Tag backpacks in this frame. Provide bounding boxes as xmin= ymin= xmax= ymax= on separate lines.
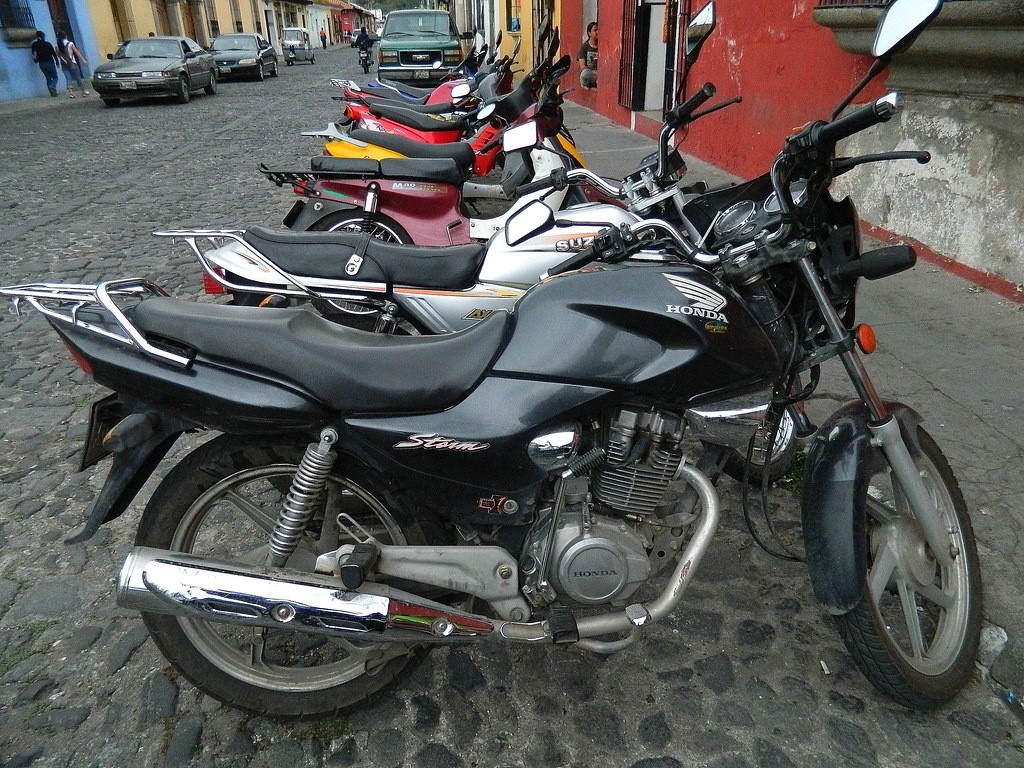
xmin=57 ymin=41 xmax=73 ymax=67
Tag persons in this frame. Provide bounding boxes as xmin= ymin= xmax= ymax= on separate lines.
xmin=576 ymin=22 xmax=598 ymax=88
xmin=320 ymin=28 xmax=327 ymax=49
xmin=345 ymin=29 xmax=352 ymax=43
xmin=31 ymin=31 xmax=59 ymax=97
xmin=54 ymin=29 xmax=89 ymax=98
xmin=356 ymin=26 xmax=374 ymax=64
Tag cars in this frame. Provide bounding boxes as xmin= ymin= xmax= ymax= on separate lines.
xmin=91 ymin=36 xmax=219 ymax=108
xmin=203 ymin=32 xmax=279 ymax=82
xmin=351 ymin=29 xmax=368 ymax=48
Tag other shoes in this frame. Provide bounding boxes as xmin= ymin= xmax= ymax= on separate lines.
xmin=83 ymin=91 xmax=89 ymax=97
xmin=50 ymin=90 xmax=59 ymax=97
xmin=70 ymin=94 xmax=76 ymax=98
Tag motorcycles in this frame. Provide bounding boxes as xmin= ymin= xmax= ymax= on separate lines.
xmin=278 ymin=27 xmax=315 ymax=66
xmin=150 ymin=1 xmax=802 ymax=488
xmin=355 ymin=42 xmax=375 ymax=74
xmin=0 ymin=0 xmax=983 ymax=718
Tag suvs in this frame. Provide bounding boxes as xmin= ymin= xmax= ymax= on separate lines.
xmin=368 ymin=8 xmax=474 ymax=87
